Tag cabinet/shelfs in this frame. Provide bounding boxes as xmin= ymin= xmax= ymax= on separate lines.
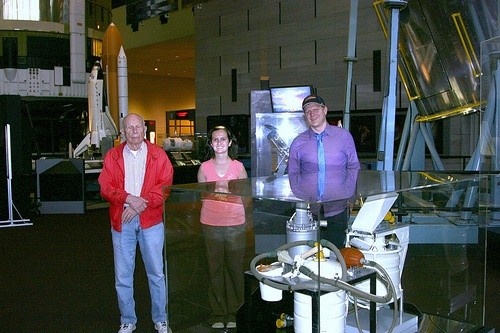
xmin=35 ymin=157 xmax=109 ymax=215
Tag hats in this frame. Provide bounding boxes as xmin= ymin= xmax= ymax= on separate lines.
xmin=302 ymin=95 xmax=325 ymax=109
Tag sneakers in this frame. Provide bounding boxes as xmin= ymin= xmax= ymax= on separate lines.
xmin=155 ymin=322 xmax=172 ymax=333
xmin=118 ymin=321 xmax=136 ymax=333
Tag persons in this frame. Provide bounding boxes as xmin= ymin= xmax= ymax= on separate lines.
xmin=98 ymin=112 xmax=174 ymax=333
xmin=198 ymin=125 xmax=248 ymax=328
xmin=288 ymin=94 xmax=361 ymax=250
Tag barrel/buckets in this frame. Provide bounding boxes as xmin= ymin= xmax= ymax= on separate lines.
xmin=256 ymin=265 xmax=282 ymax=301
xmin=285 ymin=202 xmax=318 ymax=260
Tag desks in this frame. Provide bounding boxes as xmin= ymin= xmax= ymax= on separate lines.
xmin=244 ymin=260 xmax=376 ymax=333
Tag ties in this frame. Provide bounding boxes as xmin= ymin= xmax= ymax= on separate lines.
xmin=315 ymin=133 xmax=328 ymax=196
